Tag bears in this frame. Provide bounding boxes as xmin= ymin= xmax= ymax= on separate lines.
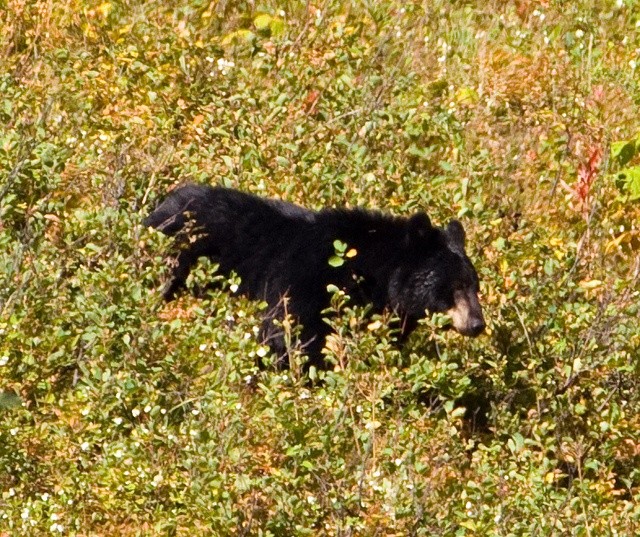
xmin=142 ymin=183 xmax=487 ymax=389
